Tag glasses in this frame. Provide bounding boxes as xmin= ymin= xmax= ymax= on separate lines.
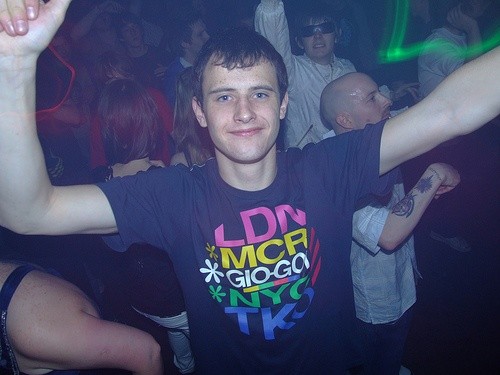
xmin=297 ymin=21 xmax=337 ymax=38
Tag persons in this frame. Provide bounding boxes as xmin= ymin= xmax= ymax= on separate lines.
xmin=67 ymin=0 xmax=215 ymax=101
xmin=0 ymin=0 xmax=500 ymax=375
xmin=320 ymin=73 xmax=460 ymax=375
xmin=0 ymin=264 xmax=162 ymax=375
xmin=254 ymin=1 xmax=421 ymax=150
xmin=416 ymin=0 xmax=487 ymax=101
xmin=92 ymin=79 xmax=195 ymax=375
xmin=169 ymin=69 xmax=213 ymax=168
